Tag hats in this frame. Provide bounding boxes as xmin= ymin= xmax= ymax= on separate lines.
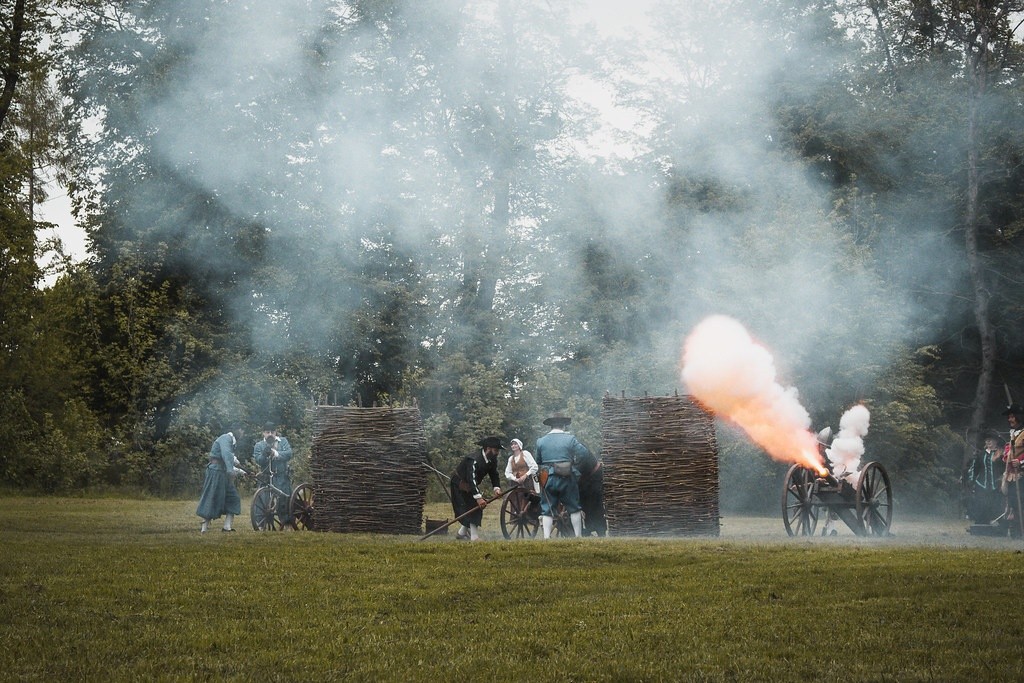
xmin=542 ymin=413 xmax=572 ymax=426
xmin=474 ymin=437 xmax=506 ymax=451
xmin=977 ymin=429 xmax=1006 ymax=448
xmin=263 ymin=421 xmax=277 ymax=431
xmin=1002 ymin=404 xmax=1024 ymax=416
xmin=817 ymin=427 xmax=833 ymax=449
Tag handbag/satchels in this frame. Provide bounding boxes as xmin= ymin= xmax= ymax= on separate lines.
xmin=554 ymin=462 xmax=571 ymax=478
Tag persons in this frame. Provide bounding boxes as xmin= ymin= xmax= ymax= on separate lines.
xmin=789 ymin=423 xmax=839 ymax=537
xmin=964 ymin=403 xmax=1024 ymax=540
xmin=196 ymin=421 xmax=293 ymax=533
xmin=449 ymin=415 xmax=604 ymax=540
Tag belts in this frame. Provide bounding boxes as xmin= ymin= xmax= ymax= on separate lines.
xmin=585 ymin=461 xmax=601 ymax=475
xmin=451 ymin=468 xmax=471 ymax=492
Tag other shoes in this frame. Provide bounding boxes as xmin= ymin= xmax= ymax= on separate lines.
xmin=456 ymin=532 xmax=469 ymax=540
xmin=822 ymin=526 xmax=826 ymax=535
xmin=832 ymin=530 xmax=837 ymax=535
xmin=221 ymin=527 xmax=236 ymax=531
